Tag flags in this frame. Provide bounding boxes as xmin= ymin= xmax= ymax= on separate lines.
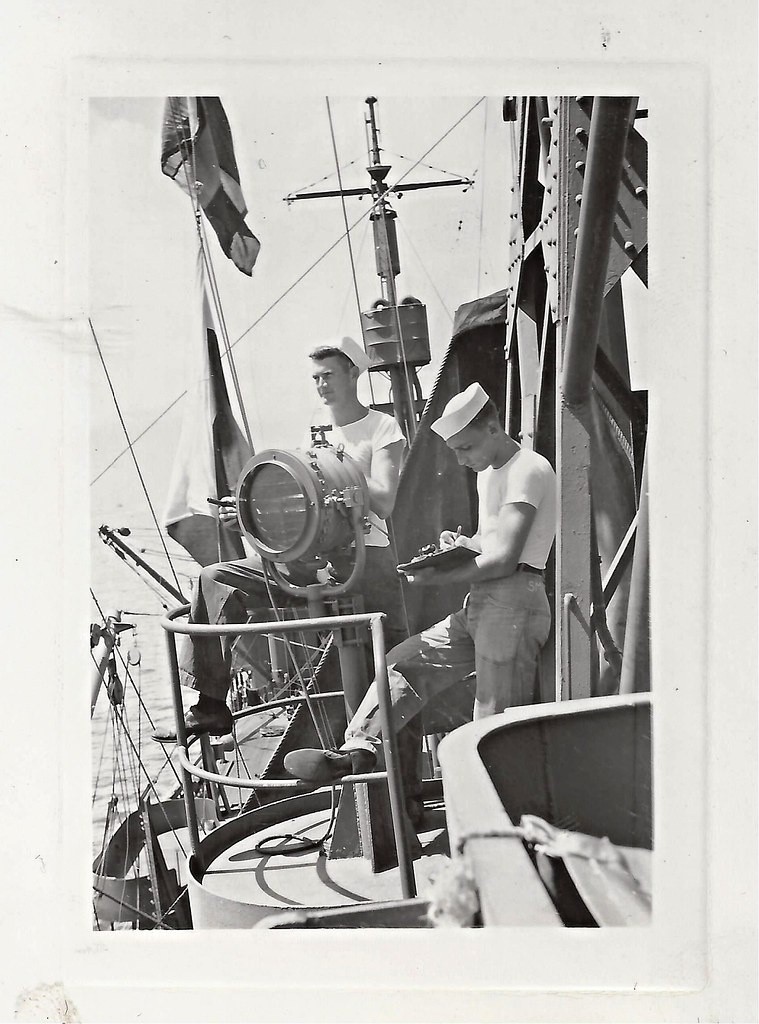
xmin=164 ymin=255 xmax=248 ymax=569
xmin=161 ymin=96 xmax=260 ymax=277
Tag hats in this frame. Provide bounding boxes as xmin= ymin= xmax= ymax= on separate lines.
xmin=317 ymin=335 xmax=371 ymax=377
xmin=429 ymin=382 xmax=490 ymax=440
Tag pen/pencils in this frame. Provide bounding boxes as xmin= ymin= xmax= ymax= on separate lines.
xmin=456 ymin=525 xmax=462 ymax=539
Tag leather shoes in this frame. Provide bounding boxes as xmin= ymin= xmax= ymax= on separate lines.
xmin=150 ymin=705 xmax=234 ymax=743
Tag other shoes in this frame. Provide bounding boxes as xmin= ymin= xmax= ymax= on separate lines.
xmin=284 ymin=749 xmax=377 ymax=781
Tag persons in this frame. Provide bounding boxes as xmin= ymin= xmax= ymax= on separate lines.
xmin=284 ymin=381 xmax=556 ymax=779
xmin=152 ymin=335 xmax=423 ymax=828
